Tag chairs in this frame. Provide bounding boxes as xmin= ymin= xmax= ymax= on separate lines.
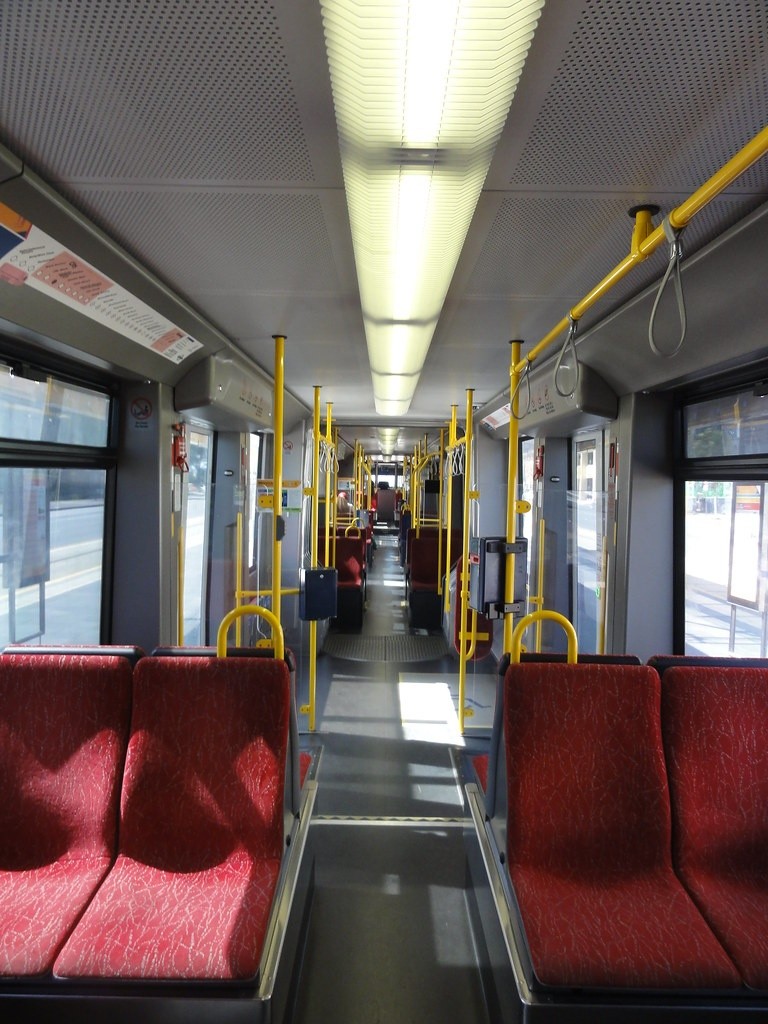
xmin=399 ymin=510 xmax=448 ymax=592
xmin=318 ymin=508 xmax=372 ymax=590
xmin=0 ymin=643 xmax=312 ymax=996
xmin=467 ymin=646 xmax=768 ymax=1003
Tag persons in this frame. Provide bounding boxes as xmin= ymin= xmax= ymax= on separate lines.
xmin=336 ymin=492 xmax=356 ymax=513
